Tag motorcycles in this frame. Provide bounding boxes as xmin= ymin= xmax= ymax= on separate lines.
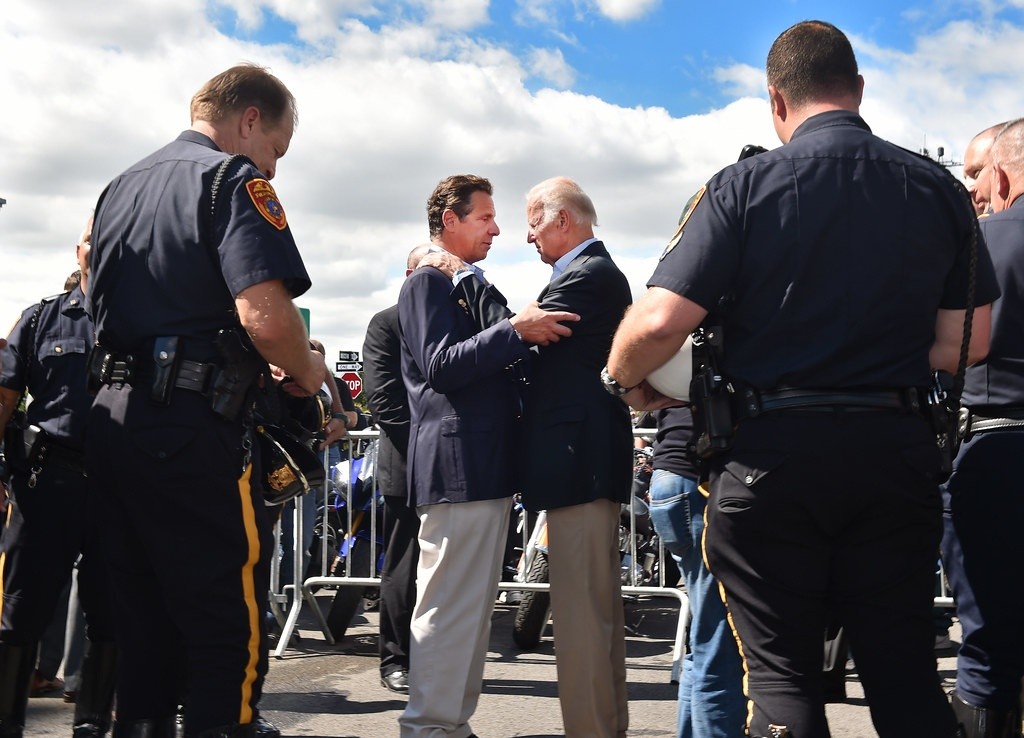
xmin=311 ymin=411 xmax=682 ymax=650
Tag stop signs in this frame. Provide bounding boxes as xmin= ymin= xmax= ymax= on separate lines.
xmin=342 ymin=373 xmax=363 ymax=399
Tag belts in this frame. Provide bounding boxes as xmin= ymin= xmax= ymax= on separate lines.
xmin=757 ymin=385 xmax=909 ymax=414
xmin=109 ymin=360 xmax=206 ymax=397
xmin=970 ymin=407 xmax=1024 ymax=433
xmin=49 ymin=447 xmax=90 ymax=477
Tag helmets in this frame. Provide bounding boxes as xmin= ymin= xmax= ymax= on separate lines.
xmin=259 ymin=424 xmax=326 ymax=507
xmin=280 ymin=376 xmax=334 ymax=435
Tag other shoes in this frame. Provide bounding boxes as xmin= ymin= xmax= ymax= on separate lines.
xmin=63 ymin=673 xmax=82 ymax=702
xmin=28 ymin=669 xmax=65 ymax=698
xmin=319 ymin=630 xmax=362 ymax=654
xmin=506 ymin=590 xmax=523 ymax=604
xmin=266 ymin=626 xmax=280 ymax=649
xmin=256 ymin=717 xmax=280 ymax=737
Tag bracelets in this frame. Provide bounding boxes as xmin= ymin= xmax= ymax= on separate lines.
xmin=333 ymin=402 xmax=343 ymax=406
xmin=513 ymin=329 xmax=523 ymax=341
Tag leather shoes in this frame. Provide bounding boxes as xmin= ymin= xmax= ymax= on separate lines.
xmin=381 ymin=669 xmax=409 ymax=694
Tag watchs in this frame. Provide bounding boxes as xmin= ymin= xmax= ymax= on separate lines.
xmin=600 ymin=366 xmax=646 ymax=397
xmin=452 ymin=267 xmax=473 ymax=279
xmin=331 ymin=414 xmax=349 ymax=428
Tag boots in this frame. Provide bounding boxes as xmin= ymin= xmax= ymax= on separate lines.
xmin=72 ymin=641 xmax=119 ymax=738
xmin=112 ymin=714 xmax=185 ymax=738
xmin=0 ymin=639 xmax=38 ymax=738
xmin=952 ymin=694 xmax=1023 ymax=738
xmin=193 ymin=721 xmax=256 ymax=738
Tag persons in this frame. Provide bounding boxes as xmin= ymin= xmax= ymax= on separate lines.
xmin=602 ymin=19 xmax=1023 ymax=738
xmin=363 ymin=175 xmax=634 ymax=738
xmin=0 ymin=66 xmax=362 ymax=738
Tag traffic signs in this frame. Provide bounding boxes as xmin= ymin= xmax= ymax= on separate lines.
xmin=335 ymin=351 xmax=363 ymax=372
